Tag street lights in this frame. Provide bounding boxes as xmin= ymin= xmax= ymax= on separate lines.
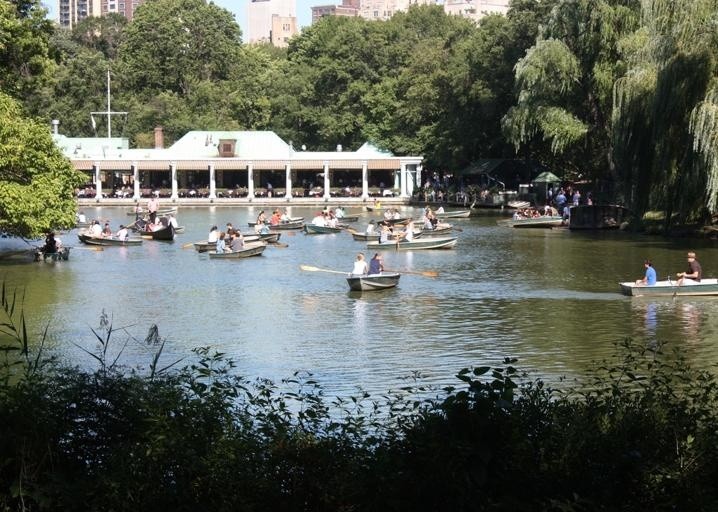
xmin=247 ymin=162 xmax=256 ymax=199
xmin=131 ymin=162 xmax=141 ymax=200
xmin=93 ymin=162 xmax=103 ymax=198
xmin=400 ymin=161 xmax=410 ymax=198
xmin=169 ymin=162 xmax=179 ymax=199
xmin=360 ymin=161 xmax=370 ymax=199
xmin=207 ymin=162 xmax=217 ymax=199
xmin=322 ymin=161 xmax=331 ymax=198
xmin=284 ymin=162 xmax=293 ymax=198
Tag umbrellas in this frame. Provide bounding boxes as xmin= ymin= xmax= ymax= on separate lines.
xmin=532 ymin=171 xmax=561 ymax=204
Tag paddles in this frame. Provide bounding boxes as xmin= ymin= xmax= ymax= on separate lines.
xmin=128 ymin=235 xmax=153 ymax=240
xmin=383 ymin=268 xmax=438 ymax=278
xmin=300 ymin=264 xmax=352 ymax=274
xmin=244 ymin=244 xmax=289 ymax=248
xmin=339 ymin=227 xmax=356 ymax=234
xmin=126 ymin=218 xmax=140 ymax=229
xmin=60 ymin=246 xmax=104 ymax=251
xmin=672 ymin=278 xmax=684 ymax=297
xmin=184 ymin=239 xmax=208 ymax=248
xmin=271 ymin=231 xmax=296 ymax=236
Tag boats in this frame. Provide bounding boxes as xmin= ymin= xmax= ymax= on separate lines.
xmin=75 ymin=221 xmax=94 ymax=228
xmin=623 ymin=295 xmax=718 ymax=309
xmin=135 ymin=217 xmax=175 ymax=241
xmin=332 ymin=214 xmax=360 ymax=222
xmin=366 ymin=206 xmax=394 ymax=211
xmin=174 ymin=227 xmax=185 ymax=235
xmin=242 ymin=233 xmax=281 ymax=242
xmin=434 ymin=210 xmax=471 ymax=218
xmin=494 ymin=217 xmax=563 ymax=229
xmin=208 ymin=244 xmax=267 ymax=259
xmin=249 ymin=214 xmax=305 ymax=229
xmin=351 ymin=218 xmax=459 ymax=249
xmin=127 ymin=206 xmax=179 ymax=218
xmin=77 ymin=234 xmax=144 ymax=247
xmin=303 ymin=222 xmax=350 ymax=234
xmin=617 ymin=278 xmax=718 ymax=297
xmin=34 ymin=247 xmax=71 ymax=263
xmin=194 ymin=235 xmax=262 ymax=251
xmin=345 ymin=273 xmax=400 ymax=291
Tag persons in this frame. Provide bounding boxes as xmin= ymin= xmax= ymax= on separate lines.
xmin=634 ymin=258 xmax=656 ymax=285
xmin=353 ymin=253 xmax=369 ymax=276
xmin=202 ymin=182 xmax=274 ymax=198
xmin=41 ymin=232 xmax=63 ymax=255
xmin=676 ymin=251 xmax=701 ymax=286
xmin=77 ymin=183 xmax=156 ymax=198
xmin=78 ymin=212 xmax=130 ymax=241
xmin=412 ymin=181 xmax=593 ymax=220
xmin=134 ymin=197 xmax=178 ymax=232
xmin=312 ymin=197 xmax=445 ymax=243
xmin=309 ymin=181 xmax=384 ymax=197
xmin=369 ymin=251 xmax=385 ymax=274
xmin=208 ymin=208 xmax=294 ymax=254
